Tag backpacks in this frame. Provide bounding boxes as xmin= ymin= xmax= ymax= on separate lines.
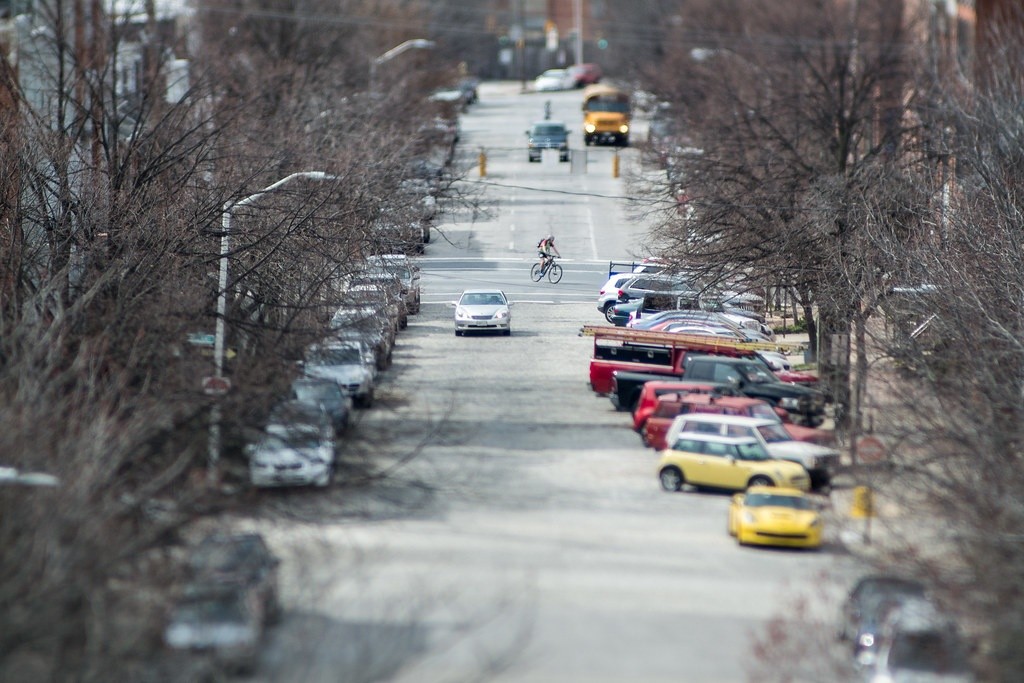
xmin=537 ymin=238 xmax=545 ymax=247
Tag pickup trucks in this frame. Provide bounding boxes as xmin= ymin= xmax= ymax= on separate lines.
xmin=607 ymin=352 xmax=826 ymax=416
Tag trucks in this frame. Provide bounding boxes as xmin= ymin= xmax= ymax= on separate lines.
xmin=580 ymin=83 xmax=636 ymax=148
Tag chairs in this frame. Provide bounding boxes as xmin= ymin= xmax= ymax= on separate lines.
xmin=487 ymin=296 xmax=503 ymax=304
xmin=464 ymin=296 xmax=476 ymax=303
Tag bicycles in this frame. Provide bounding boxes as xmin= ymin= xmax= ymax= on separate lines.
xmin=530 ymin=252 xmax=563 ymax=284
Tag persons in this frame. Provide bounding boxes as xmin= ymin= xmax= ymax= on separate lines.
xmin=538 ymin=234 xmax=560 ymax=277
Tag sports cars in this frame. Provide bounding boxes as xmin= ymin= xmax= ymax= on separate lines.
xmin=728 ymin=485 xmax=830 ymax=551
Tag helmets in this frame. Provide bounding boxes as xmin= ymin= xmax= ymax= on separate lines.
xmin=547 ymin=235 xmax=554 ymax=240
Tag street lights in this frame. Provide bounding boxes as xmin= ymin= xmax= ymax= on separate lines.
xmin=368 ymin=38 xmax=438 ymax=89
xmin=205 ymin=170 xmax=336 ymax=477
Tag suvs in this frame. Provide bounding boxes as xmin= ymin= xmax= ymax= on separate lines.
xmin=525 ymin=122 xmax=573 ymax=163
xmin=295 ymin=341 xmax=375 ymax=409
xmin=632 ymin=378 xmax=794 ymax=442
xmin=645 ymin=393 xmax=831 ymax=454
xmin=664 ymin=412 xmax=842 ymax=488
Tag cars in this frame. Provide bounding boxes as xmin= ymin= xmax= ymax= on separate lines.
xmin=533 ymin=62 xmax=602 ymax=92
xmin=588 ymin=253 xmax=820 ymax=405
xmin=339 ymin=75 xmax=477 ymax=335
xmin=322 ymin=305 xmax=394 ymax=371
xmin=834 ymin=571 xmax=978 ymax=683
xmin=450 ymin=288 xmax=515 ymax=337
xmin=655 ymin=431 xmax=813 ymax=497
xmin=258 ymin=398 xmax=340 ymax=445
xmin=157 ymin=531 xmax=285 ymax=677
xmin=242 ymin=423 xmax=336 ymax=490
xmin=880 ymin=263 xmax=947 ymax=306
xmin=274 ymin=378 xmax=353 ymax=439
